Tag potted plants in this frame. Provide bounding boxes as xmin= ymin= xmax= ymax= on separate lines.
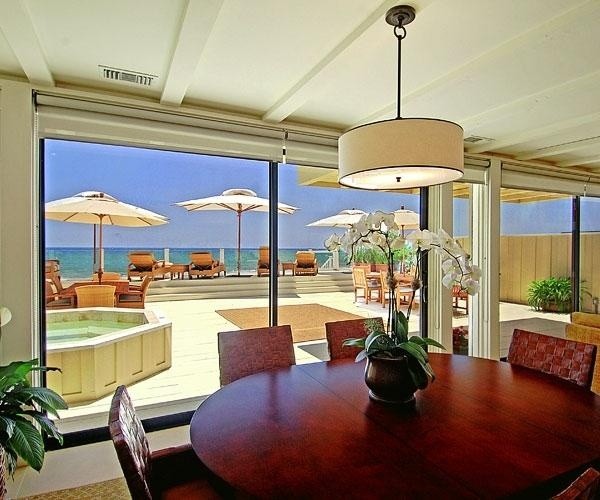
xmin=525 ymin=272 xmax=594 ymax=317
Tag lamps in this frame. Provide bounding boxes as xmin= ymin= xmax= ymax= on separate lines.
xmin=328 ymin=3 xmax=466 ymax=192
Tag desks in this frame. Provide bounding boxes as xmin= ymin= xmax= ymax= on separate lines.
xmin=169 ymin=264 xmax=190 ymax=281
xmin=281 ymin=262 xmax=295 ymax=277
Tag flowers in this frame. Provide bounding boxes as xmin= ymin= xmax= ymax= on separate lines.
xmin=316 ymin=205 xmax=484 ymax=392
xmin=0 ymin=303 xmax=71 ymax=486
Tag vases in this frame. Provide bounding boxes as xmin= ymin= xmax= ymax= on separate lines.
xmin=360 ymin=345 xmax=421 ymax=409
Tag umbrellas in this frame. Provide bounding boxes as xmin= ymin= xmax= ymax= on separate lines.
xmin=169 ymin=189 xmax=301 ymax=276
xmin=305 ymin=205 xmax=420 ymax=301
xmin=46 ymin=190 xmax=172 ymax=285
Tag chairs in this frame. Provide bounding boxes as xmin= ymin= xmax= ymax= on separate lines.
xmin=103 ymin=382 xmax=246 ymax=500
xmin=353 ymin=260 xmax=423 ymax=314
xmin=186 ymin=250 xmax=227 ymax=282
xmin=540 ymin=465 xmax=600 ymax=500
xmin=504 ymin=327 xmax=598 ymax=394
xmin=125 ymin=249 xmax=161 ymax=282
xmin=255 ymin=245 xmax=273 ymax=278
xmin=45 ymin=257 xmax=60 ymax=272
xmin=45 ymin=268 xmax=153 ymax=312
xmin=214 ymin=322 xmax=296 ymax=390
xmin=295 ymin=251 xmax=318 ymax=276
xmin=563 ymin=310 xmax=600 ymax=397
xmin=322 ymin=312 xmax=391 ymax=363
